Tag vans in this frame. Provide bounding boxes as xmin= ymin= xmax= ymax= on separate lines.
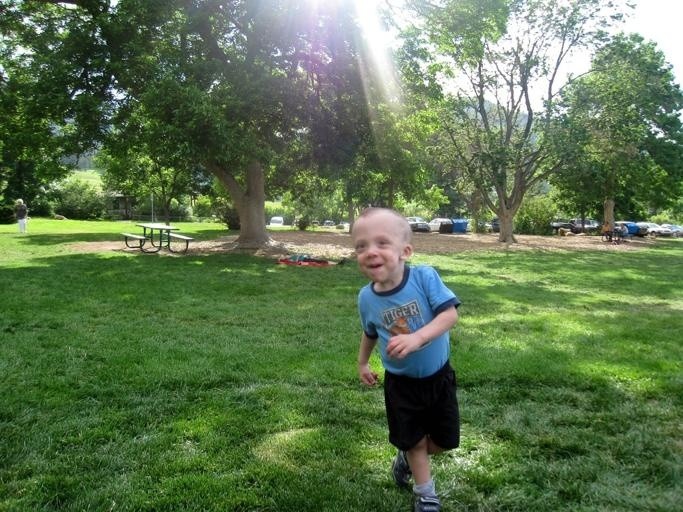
xmin=268 ymin=215 xmax=286 ymax=227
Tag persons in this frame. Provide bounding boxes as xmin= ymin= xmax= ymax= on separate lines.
xmin=348 ymin=207 xmax=461 ymax=511
xmin=617 ymin=223 xmax=628 ymax=241
xmin=602 ymin=221 xmax=612 ymax=242
xmin=13 ymin=198 xmax=28 ymax=234
xmin=279 ymin=253 xmax=346 ymax=267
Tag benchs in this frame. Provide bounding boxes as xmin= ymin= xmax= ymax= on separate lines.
xmin=120 ymin=223 xmax=195 ymax=253
xmin=603 ymin=230 xmax=633 ymax=242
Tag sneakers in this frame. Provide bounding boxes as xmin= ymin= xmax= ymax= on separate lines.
xmin=390 ymin=448 xmax=412 ymax=486
xmin=415 ymin=492 xmax=440 ymax=511
